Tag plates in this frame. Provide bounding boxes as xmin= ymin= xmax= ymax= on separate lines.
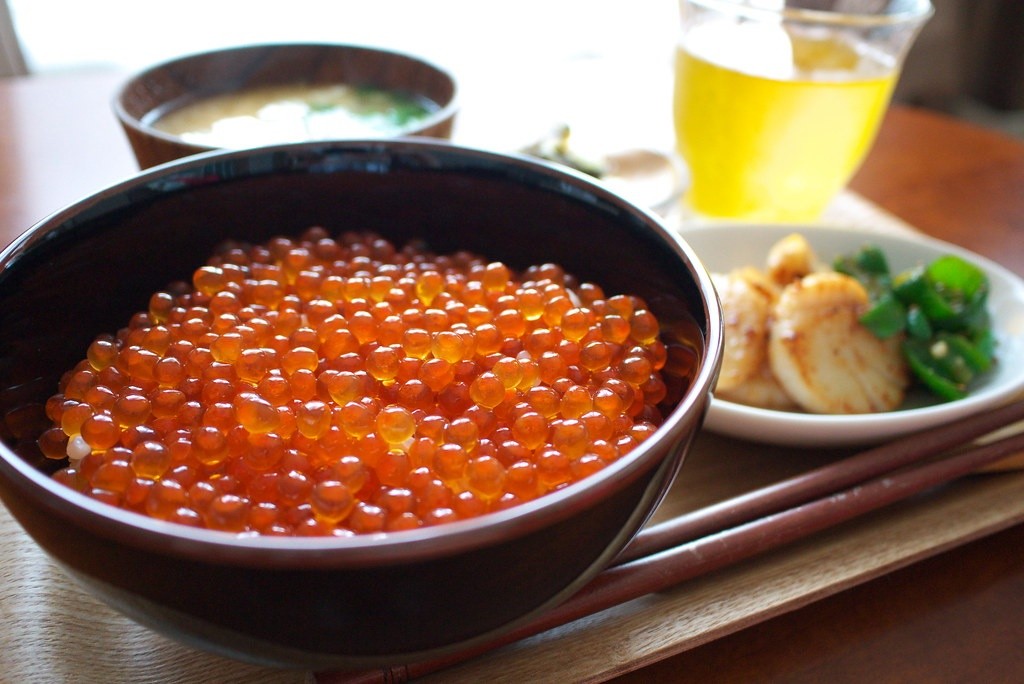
xmin=675 ymin=223 xmax=1023 ymax=445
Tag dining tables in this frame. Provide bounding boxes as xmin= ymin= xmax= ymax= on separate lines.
xmin=1 ymin=60 xmax=1024 ymax=684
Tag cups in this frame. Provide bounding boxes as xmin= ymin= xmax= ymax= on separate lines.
xmin=672 ymin=1 xmax=935 ymax=222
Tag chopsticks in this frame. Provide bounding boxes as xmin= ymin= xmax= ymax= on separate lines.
xmin=311 ymin=394 xmax=1024 ymax=684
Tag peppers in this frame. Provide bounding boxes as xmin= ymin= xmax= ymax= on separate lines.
xmin=834 ymin=245 xmax=993 ymax=399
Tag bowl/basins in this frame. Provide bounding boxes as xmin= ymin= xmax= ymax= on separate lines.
xmin=0 ymin=137 xmax=723 ymax=669
xmin=113 ymin=42 xmax=460 ymax=173
